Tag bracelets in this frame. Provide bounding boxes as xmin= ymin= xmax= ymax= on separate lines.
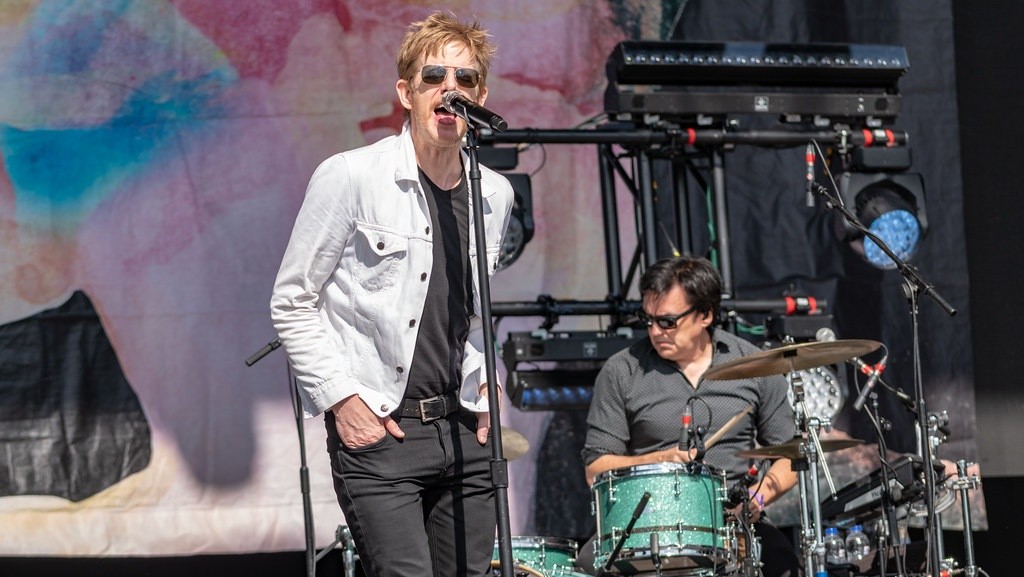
xmin=749 ymin=489 xmax=765 ymax=512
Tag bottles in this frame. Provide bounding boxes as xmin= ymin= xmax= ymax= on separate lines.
xmin=846 ymin=525 xmax=869 ymax=577
xmin=824 ymin=528 xmax=846 ymax=564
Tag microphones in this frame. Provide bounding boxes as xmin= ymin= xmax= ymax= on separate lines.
xmin=724 ymin=459 xmax=765 ymax=509
xmin=816 ymin=327 xmax=875 ymax=378
xmin=679 ymin=403 xmax=692 ymax=452
xmin=442 ymin=90 xmax=508 ymax=133
xmin=853 ymin=355 xmax=888 ymax=411
xmin=804 ymin=142 xmax=816 ymax=208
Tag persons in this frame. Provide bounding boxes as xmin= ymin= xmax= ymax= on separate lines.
xmin=574 ymin=254 xmax=799 ymax=577
xmin=270 ymin=8 xmax=514 ymax=577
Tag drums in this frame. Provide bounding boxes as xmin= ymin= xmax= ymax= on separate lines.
xmin=591 ymin=461 xmax=729 ymax=577
xmin=491 ymin=538 xmax=579 ymax=576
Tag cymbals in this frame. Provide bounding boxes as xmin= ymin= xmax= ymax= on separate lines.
xmin=737 ymin=436 xmax=865 ymax=460
xmin=489 ymin=426 xmax=530 ymax=462
xmin=699 ymin=339 xmax=883 ymax=382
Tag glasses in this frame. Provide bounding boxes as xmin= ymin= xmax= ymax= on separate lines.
xmin=638 ymin=306 xmax=697 ymax=329
xmin=417 ymin=65 xmax=482 ymax=88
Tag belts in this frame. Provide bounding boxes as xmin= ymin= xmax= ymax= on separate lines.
xmin=391 ymin=389 xmax=460 ymax=423
